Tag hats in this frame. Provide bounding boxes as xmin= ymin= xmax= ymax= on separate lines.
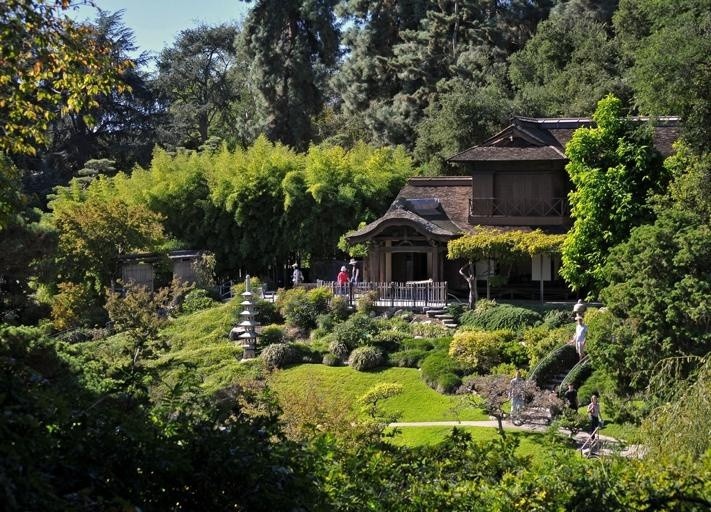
xmin=340 ymin=266 xmax=346 ymax=271
xmin=348 ymin=259 xmax=357 ymax=264
xmin=291 ymin=264 xmax=297 ymax=267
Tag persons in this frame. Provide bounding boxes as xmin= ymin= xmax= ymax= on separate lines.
xmin=349 ymin=259 xmax=360 ymax=285
xmin=587 ymin=395 xmax=603 ymax=441
xmin=291 ymin=263 xmax=305 ymax=284
xmin=509 ymin=371 xmax=525 ymax=420
xmin=550 ymin=385 xmax=562 ymax=420
xmin=568 ymin=313 xmax=588 ymax=360
xmin=565 ymin=382 xmax=580 ymax=414
xmin=338 ymin=266 xmax=351 ymax=285
